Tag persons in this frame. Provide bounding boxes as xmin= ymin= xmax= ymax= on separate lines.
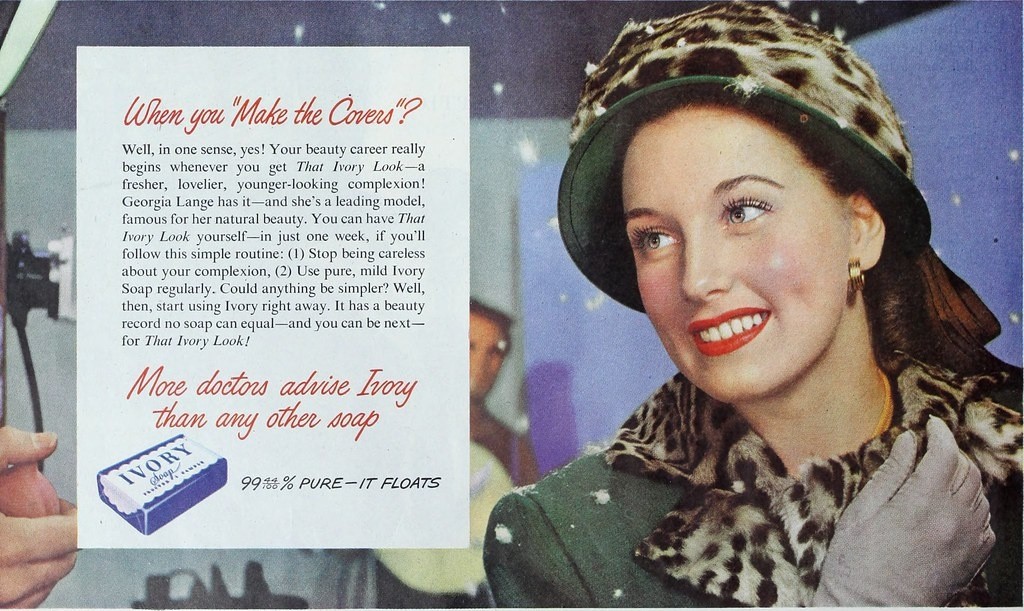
xmin=483 ymin=0 xmax=1023 ymax=608
xmin=298 ymin=300 xmax=534 ymax=609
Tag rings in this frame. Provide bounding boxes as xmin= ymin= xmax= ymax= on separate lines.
xmin=8 ymin=465 xmax=13 ymax=475
